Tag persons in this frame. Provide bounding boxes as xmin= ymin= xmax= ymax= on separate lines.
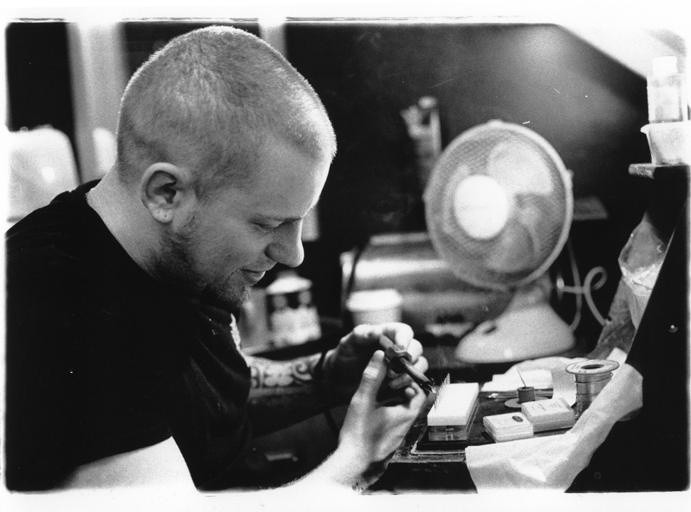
xmin=6 ymin=23 xmax=434 ymax=494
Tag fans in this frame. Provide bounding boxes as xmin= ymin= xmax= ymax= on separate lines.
xmin=421 ymin=118 xmax=588 ymax=364
